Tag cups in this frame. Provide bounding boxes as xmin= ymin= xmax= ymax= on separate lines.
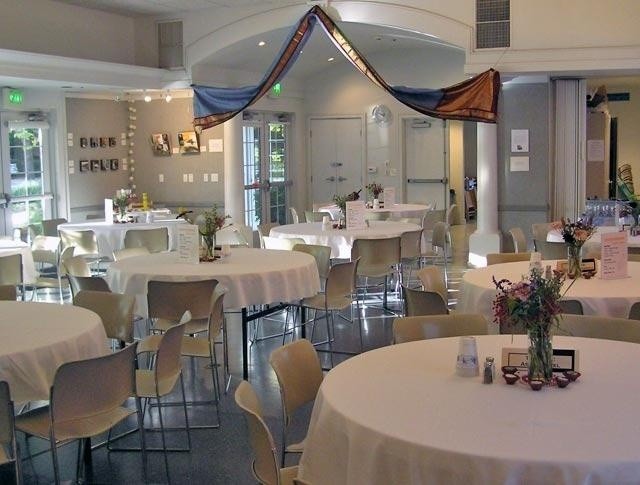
xmin=146 ymin=210 xmax=152 ymax=225
xmin=560 ymin=269 xmax=597 ymax=279
xmin=221 ymin=242 xmax=231 ymax=257
xmin=142 ymin=192 xmax=148 ymax=208
xmin=502 ymin=365 xmax=581 ymax=390
xmin=323 ymin=216 xmax=330 ymax=226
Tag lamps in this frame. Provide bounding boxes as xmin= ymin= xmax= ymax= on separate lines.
xmin=110 ymin=87 xmax=174 ymax=104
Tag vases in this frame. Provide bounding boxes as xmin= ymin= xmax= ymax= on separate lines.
xmin=526 ymin=329 xmax=554 ymax=386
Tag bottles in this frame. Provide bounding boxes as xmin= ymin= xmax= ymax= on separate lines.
xmin=482 ymin=357 xmax=497 ymax=384
xmin=455 ymin=336 xmax=479 ymax=377
xmin=332 ymin=218 xmax=346 ymax=229
xmin=545 ymin=265 xmax=552 ymax=280
xmin=366 ymin=198 xmax=380 ymax=209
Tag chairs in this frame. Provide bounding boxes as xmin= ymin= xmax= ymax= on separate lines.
xmin=1 ymin=379 xmax=37 ymax=483
xmin=106 ymin=310 xmax=195 ymax=454
xmin=282 ymin=254 xmax=365 ymax=372
xmin=2 ymin=201 xmax=637 ymax=322
xmin=234 ymin=381 xmax=305 ymax=483
xmin=135 ymin=291 xmax=225 ymax=434
xmin=268 ymin=339 xmax=330 ymax=468
xmin=547 ymin=311 xmax=638 ymax=342
xmin=11 ymin=338 xmax=150 ymax=484
xmin=391 ymin=314 xmax=489 ymax=346
xmin=72 ymin=291 xmax=147 ymax=451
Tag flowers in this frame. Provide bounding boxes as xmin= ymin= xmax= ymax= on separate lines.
xmin=489 ymin=262 xmax=586 ymax=341
